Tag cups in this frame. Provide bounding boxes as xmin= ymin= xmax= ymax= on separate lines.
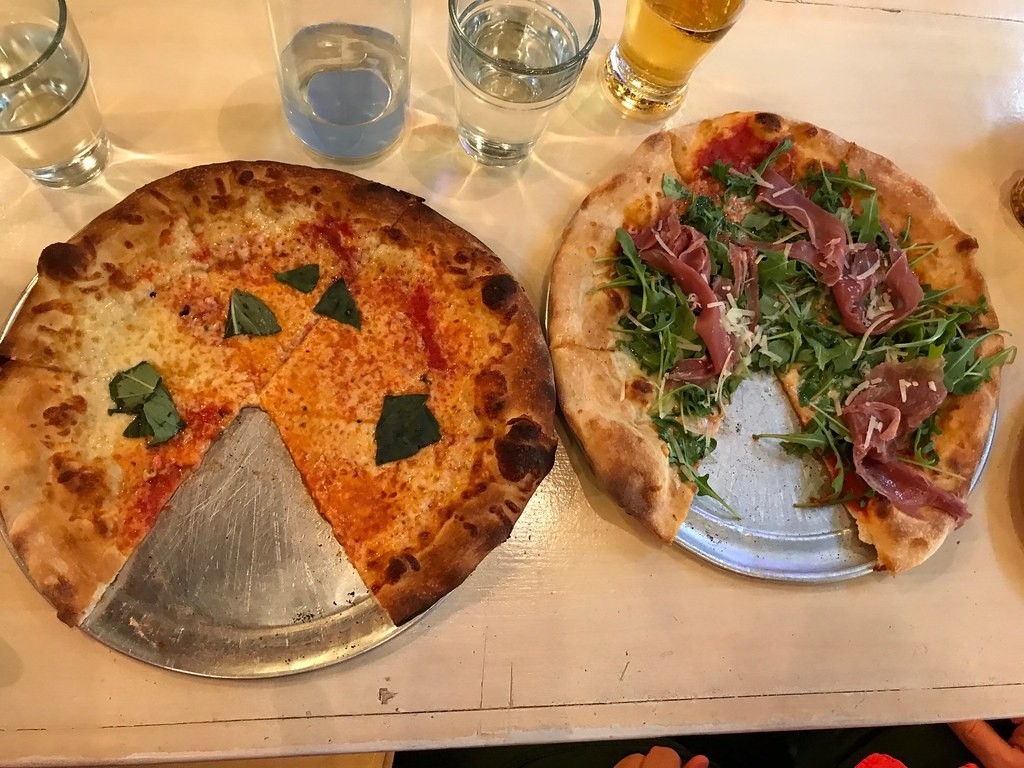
xmin=0 ymin=0 xmax=110 ymax=189
xmin=266 ymin=0 xmax=412 ymax=168
xmin=449 ymin=0 xmax=601 ymax=168
xmin=598 ymin=0 xmax=748 ymax=124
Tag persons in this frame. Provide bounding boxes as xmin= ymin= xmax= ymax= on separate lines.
xmin=385 ymin=718 xmax=1024 ymax=768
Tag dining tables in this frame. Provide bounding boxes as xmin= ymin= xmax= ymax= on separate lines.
xmin=0 ymin=1 xmax=1024 ymax=768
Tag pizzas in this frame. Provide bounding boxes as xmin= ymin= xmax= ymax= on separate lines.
xmin=548 ymin=114 xmax=1002 ymax=570
xmin=0 ymin=159 xmax=559 ymax=626
